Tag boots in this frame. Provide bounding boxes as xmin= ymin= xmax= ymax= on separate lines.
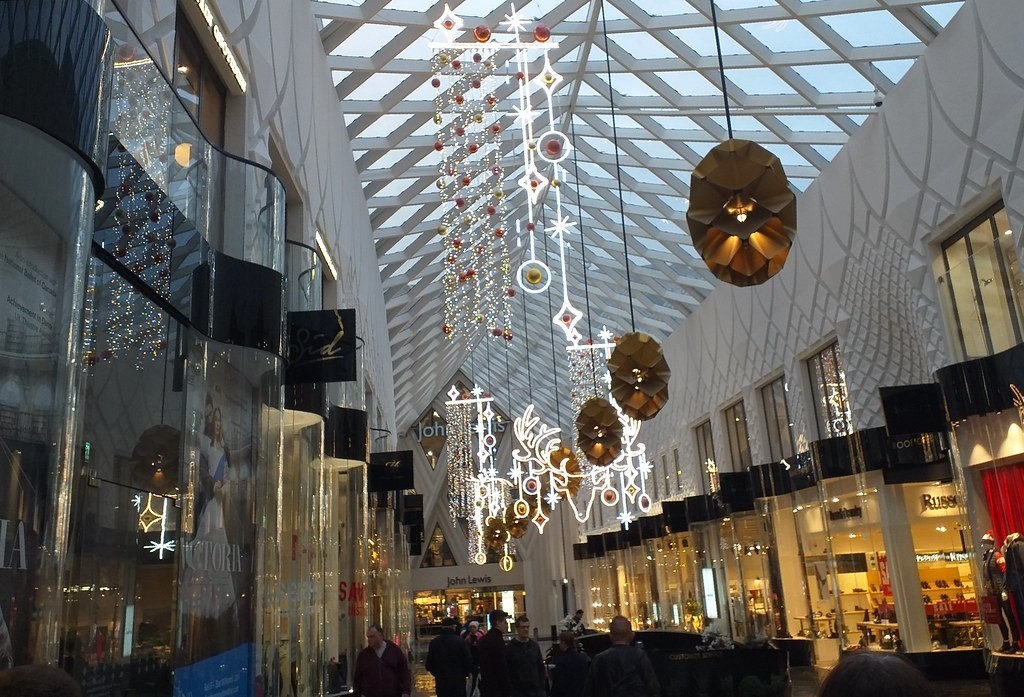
xmin=998 ymin=641 xmax=1010 ymax=652
xmin=1005 ymin=641 xmax=1019 ymax=654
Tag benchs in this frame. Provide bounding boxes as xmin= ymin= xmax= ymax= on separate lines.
xmin=857 ymin=621 xmax=899 ymax=649
xmin=793 ymin=616 xmax=837 ymax=638
xmin=948 ymin=620 xmax=982 ymax=649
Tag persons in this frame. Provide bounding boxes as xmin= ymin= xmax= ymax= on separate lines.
xmin=585 ymin=616 xmax=660 ymax=697
xmin=353 ymin=624 xmax=412 ymax=697
xmin=424 ymin=618 xmax=474 ymax=697
xmin=550 ymin=631 xmax=590 ymax=697
xmin=981 ymin=533 xmax=1021 ymax=654
xmin=458 ymin=615 xmax=485 ymax=637
xmin=1001 ymin=532 xmax=1024 ymax=655
xmin=465 ymin=621 xmax=484 ymax=697
xmin=477 ymin=610 xmax=511 ymax=697
xmin=181 ymin=406 xmax=240 ymax=647
xmin=504 ymin=615 xmax=550 ymax=697
xmin=563 ymin=609 xmax=586 ymax=636
xmin=701 ymin=616 xmax=716 ymax=636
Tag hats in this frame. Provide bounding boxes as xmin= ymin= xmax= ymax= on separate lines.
xmin=468 ymin=621 xmax=479 ymax=630
xmin=443 ymin=618 xmax=458 ymax=626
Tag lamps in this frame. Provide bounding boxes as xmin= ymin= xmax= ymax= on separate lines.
xmin=470 ymin=351 xmax=503 ymax=558
xmin=743 ymin=545 xmax=752 ymax=556
xmin=130 ymin=159 xmax=181 ymax=494
xmin=540 ymin=204 xmax=581 ymax=501
xmin=520 ymin=259 xmax=552 ymax=521
xmin=571 ymin=111 xmax=623 ymax=467
xmin=485 ymin=330 xmax=508 ymax=548
xmin=682 ymin=539 xmax=689 ymax=548
xmin=599 ymin=0 xmax=672 ymax=420
xmin=502 ymin=301 xmax=530 ymax=539
xmin=685 ymin=0 xmax=797 ymax=287
xmin=668 ymin=541 xmax=674 ymax=551
xmin=657 ymin=543 xmax=662 ymax=552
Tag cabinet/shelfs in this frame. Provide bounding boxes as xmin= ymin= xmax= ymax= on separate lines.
xmin=866 ymin=567 xmax=971 ymax=619
